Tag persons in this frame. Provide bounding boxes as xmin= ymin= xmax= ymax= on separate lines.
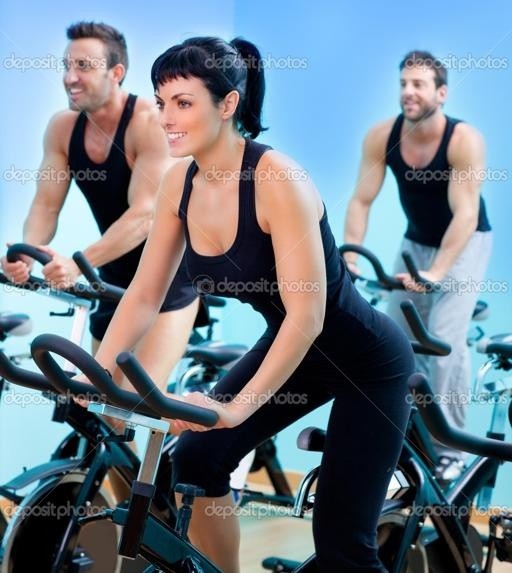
xmin=344 ymin=51 xmax=493 ymax=480
xmin=67 ymin=35 xmax=415 ymax=570
xmin=2 ymin=22 xmax=206 ymax=502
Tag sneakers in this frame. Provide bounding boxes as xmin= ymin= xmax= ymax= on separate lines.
xmin=435 ymin=456 xmax=463 ymax=481
